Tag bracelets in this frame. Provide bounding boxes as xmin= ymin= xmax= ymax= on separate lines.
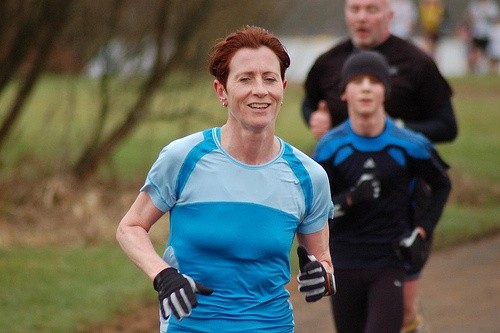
xmin=317 ymin=258 xmax=333 ymax=268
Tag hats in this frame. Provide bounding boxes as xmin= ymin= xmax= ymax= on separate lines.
xmin=338 ymin=50 xmax=392 ymax=100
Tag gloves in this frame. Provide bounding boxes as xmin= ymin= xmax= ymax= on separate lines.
xmin=395 ymin=227 xmax=423 ymax=272
xmin=152 ymin=266 xmax=215 ymax=322
xmin=295 ymin=245 xmax=329 ymax=302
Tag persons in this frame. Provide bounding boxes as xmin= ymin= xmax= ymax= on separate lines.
xmin=311 ymin=51 xmax=451 ymax=333
xmin=387 ymin=0 xmax=500 ymax=76
xmin=302 ymin=0 xmax=458 ymax=333
xmin=116 ymin=26 xmax=336 ymax=333
